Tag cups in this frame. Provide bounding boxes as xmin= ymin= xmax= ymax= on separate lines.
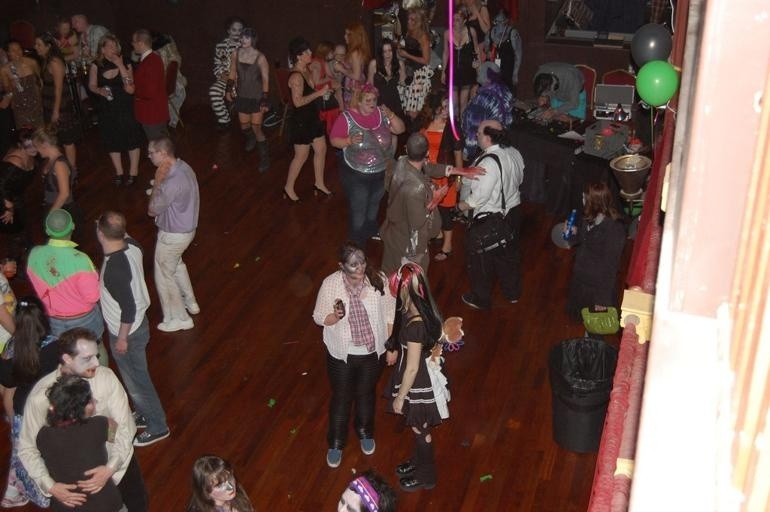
xmin=332 ymin=299 xmax=345 ymax=320
xmin=355 ymin=129 xmax=364 ymax=147
xmin=323 ymin=94 xmax=330 ymax=101
xmin=455 ymin=175 xmax=461 ymax=192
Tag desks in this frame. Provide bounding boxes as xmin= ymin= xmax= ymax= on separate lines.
xmin=509 ymin=106 xmax=616 ymax=225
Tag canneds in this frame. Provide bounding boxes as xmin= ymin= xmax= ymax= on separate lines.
xmin=103 ymin=86 xmax=113 ymax=101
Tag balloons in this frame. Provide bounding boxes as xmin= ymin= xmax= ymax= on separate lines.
xmin=635 ymin=60 xmax=678 ymax=109
xmin=630 ymin=21 xmax=673 ymax=67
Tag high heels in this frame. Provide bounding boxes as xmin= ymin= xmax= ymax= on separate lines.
xmin=282 ymin=186 xmax=304 ymax=205
xmin=313 ymin=184 xmax=334 ymax=200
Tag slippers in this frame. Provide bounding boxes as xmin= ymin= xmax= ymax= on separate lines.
xmin=433 ymin=250 xmax=454 ymax=263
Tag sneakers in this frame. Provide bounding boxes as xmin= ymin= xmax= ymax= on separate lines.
xmin=360 ymin=439 xmax=377 ymax=455
xmin=327 ymin=449 xmax=343 ymax=468
xmin=155 ymin=301 xmax=200 ymax=331
xmin=461 ymin=292 xmax=489 ymax=312
xmin=399 ymin=474 xmax=437 ymax=492
xmin=113 ymin=174 xmax=139 ymax=189
xmin=395 ymin=460 xmax=416 ymax=476
xmin=134 ymin=427 xmax=171 ymax=445
xmin=133 ymin=410 xmax=146 ymax=426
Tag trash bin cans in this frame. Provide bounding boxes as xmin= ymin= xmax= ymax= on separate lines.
xmin=548 ymin=339 xmax=618 ymax=453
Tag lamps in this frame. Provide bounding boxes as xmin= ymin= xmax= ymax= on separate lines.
xmin=609 ymin=152 xmax=653 ymax=218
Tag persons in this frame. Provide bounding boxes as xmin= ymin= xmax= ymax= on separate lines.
xmin=0 ymin=13 xmax=202 ymax=512
xmin=310 ymin=239 xmax=391 ymax=468
xmin=223 ymin=29 xmax=272 ymax=174
xmin=286 ymin=2 xmax=525 ymax=310
xmin=182 ymin=455 xmax=255 ymax=511
xmin=334 ymin=470 xmax=401 ymax=510
xmin=532 ymin=61 xmax=587 ymax=120
xmin=560 ymin=177 xmax=622 ymax=327
xmin=208 ymin=18 xmax=253 ymax=130
xmin=383 ymin=262 xmax=450 ymax=492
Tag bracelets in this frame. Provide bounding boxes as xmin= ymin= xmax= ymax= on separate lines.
xmin=396 ymin=393 xmax=407 ymax=400
xmin=261 ymin=92 xmax=271 ymax=107
xmin=225 ymin=79 xmax=235 ymax=94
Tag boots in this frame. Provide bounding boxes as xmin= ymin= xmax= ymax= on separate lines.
xmin=243 ymin=128 xmax=271 ymax=174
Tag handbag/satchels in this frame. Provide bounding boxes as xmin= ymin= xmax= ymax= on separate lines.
xmin=581 ymin=303 xmax=620 ymax=336
xmin=463 ymin=212 xmax=522 ymax=283
xmin=376 ymin=190 xmax=390 ymax=225
xmin=383 ymin=160 xmax=396 ymax=189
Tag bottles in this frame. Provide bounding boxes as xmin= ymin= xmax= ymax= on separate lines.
xmin=81 ymin=58 xmax=89 ymax=80
xmin=124 ymin=76 xmax=133 ymax=86
xmin=104 ymin=85 xmax=115 ymax=102
xmin=561 ymin=209 xmax=576 ymax=242
xmin=614 ymin=103 xmax=627 ymax=122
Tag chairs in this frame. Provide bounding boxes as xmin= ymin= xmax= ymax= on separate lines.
xmin=603 ymin=68 xmax=637 ymax=88
xmin=275 ymin=70 xmax=295 ymax=134
xmin=574 ymin=63 xmax=596 ymax=110
